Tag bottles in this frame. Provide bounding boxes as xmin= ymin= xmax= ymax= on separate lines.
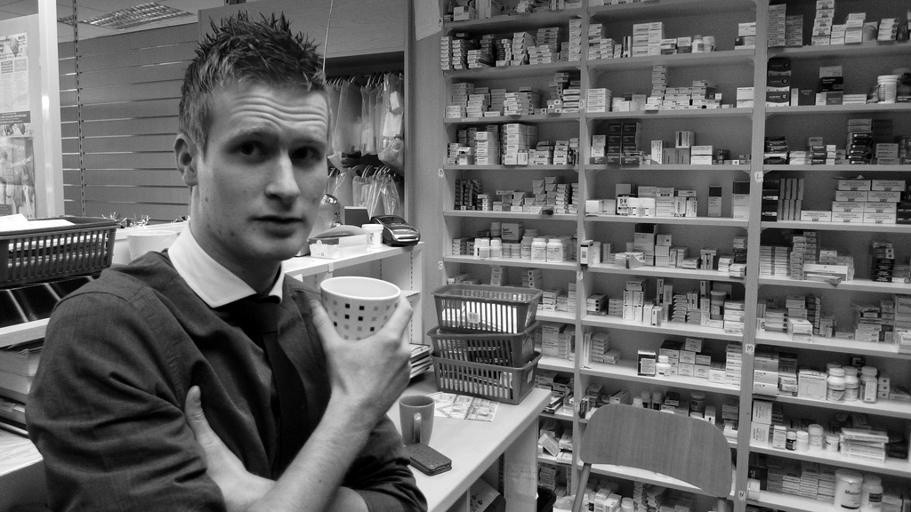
xmin=691 ymin=34 xmax=717 ymax=52
xmin=630 ymin=389 xmax=708 ymax=418
xmin=833 ymin=469 xmax=885 ymax=511
xmin=619 ymin=497 xmax=635 ymax=511
xmin=471 ymin=219 xmax=576 ymax=263
xmin=823 ymin=366 xmax=882 ymax=406
xmin=783 ymin=424 xmax=842 ymax=458
xmin=654 ymin=356 xmax=673 ymax=379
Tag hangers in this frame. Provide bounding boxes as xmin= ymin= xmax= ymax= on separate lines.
xmin=324 ymin=71 xmax=403 ymax=88
xmin=326 ymin=160 xmax=397 ymax=180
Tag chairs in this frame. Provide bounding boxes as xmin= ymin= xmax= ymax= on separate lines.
xmin=570 ymin=404 xmax=733 ymax=512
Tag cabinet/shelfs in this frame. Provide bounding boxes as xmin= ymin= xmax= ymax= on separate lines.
xmin=408 ymin=0 xmax=911 ymax=511
xmin=0 ymin=224 xmax=428 ymax=479
xmin=199 ymin=0 xmax=412 ymax=228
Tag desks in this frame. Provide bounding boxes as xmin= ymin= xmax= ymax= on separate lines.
xmin=385 ymin=371 xmax=553 ymax=511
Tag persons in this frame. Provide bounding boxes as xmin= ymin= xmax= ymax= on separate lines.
xmin=25 ymin=7 xmax=430 ymax=512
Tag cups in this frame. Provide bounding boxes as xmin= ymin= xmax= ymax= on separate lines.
xmin=399 ymin=393 xmax=435 ymax=447
xmin=316 ymin=275 xmax=401 ymax=342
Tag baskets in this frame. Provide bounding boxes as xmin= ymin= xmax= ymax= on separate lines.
xmin=431 ymin=283 xmax=544 ymax=337
xmin=429 ymin=349 xmax=544 ymax=406
xmin=425 ymin=319 xmax=542 ymax=368
xmin=0 ymin=216 xmax=120 ymax=289
